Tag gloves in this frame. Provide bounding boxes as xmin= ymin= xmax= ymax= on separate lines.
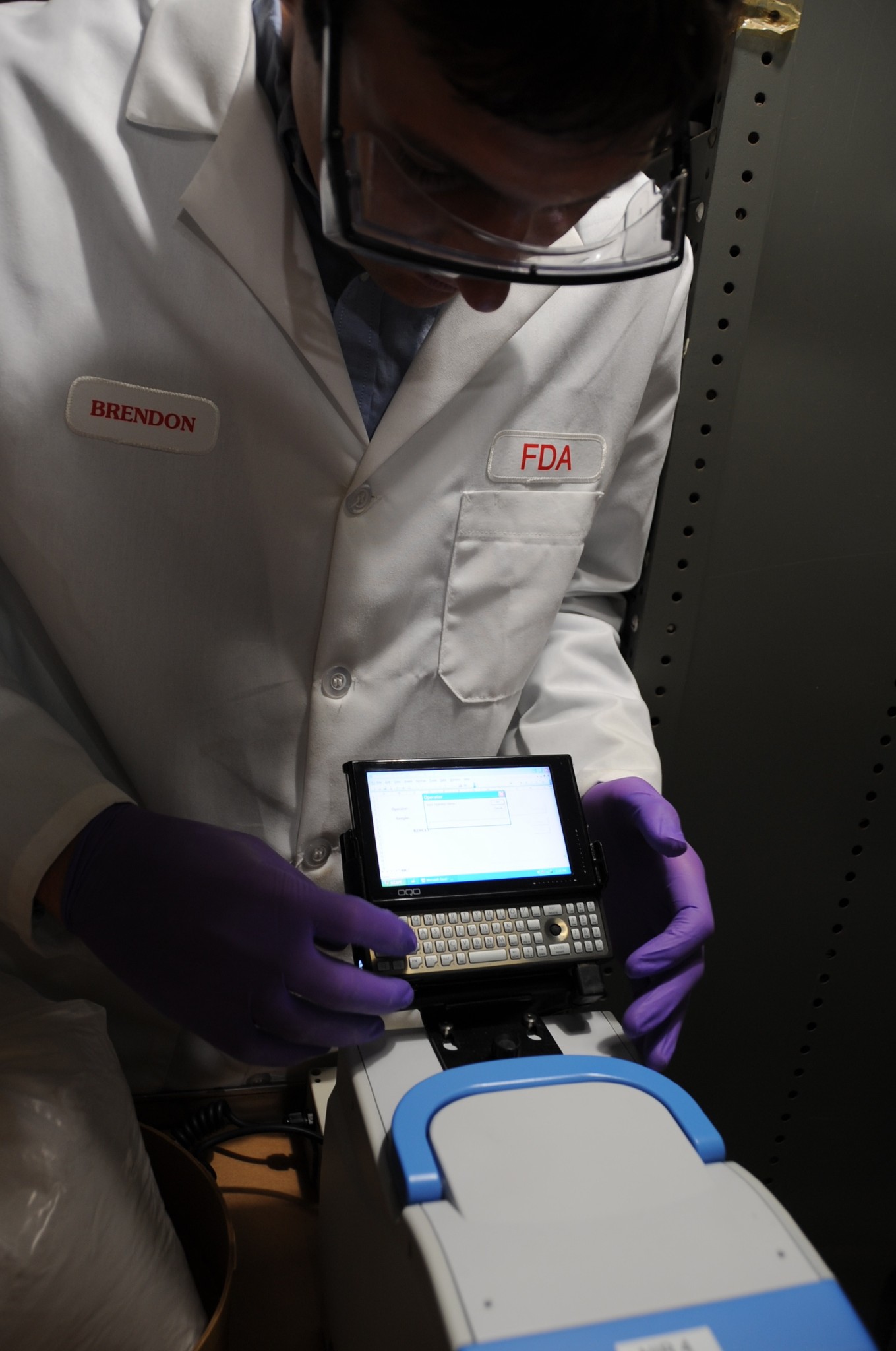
xmin=59 ymin=798 xmax=412 ymax=1065
xmin=580 ymin=778 xmax=713 ymax=1067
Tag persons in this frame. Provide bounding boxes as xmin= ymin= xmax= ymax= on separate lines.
xmin=1 ymin=0 xmax=748 ymax=1090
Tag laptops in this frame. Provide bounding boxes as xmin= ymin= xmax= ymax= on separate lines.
xmin=344 ymin=752 xmax=619 ymax=1034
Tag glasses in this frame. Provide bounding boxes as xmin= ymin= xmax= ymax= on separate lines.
xmin=315 ymin=0 xmax=691 ymax=288
